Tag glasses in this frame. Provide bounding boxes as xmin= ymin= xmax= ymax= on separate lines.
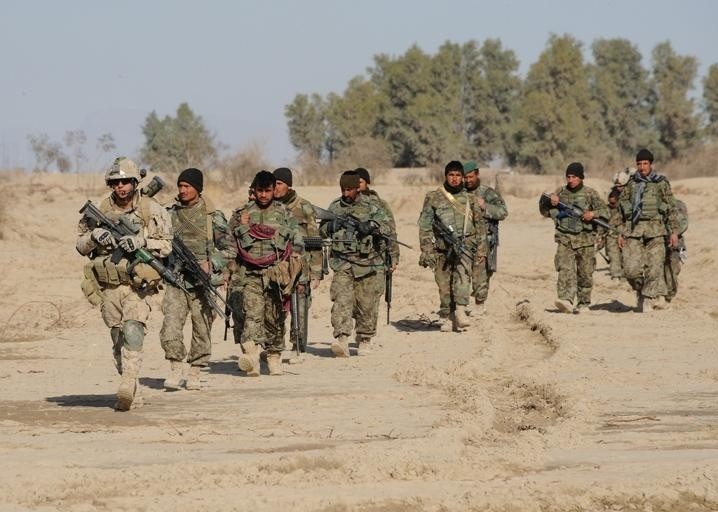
xmin=113 ymin=179 xmax=130 ymax=185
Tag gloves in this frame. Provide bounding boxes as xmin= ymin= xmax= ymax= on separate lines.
xmin=94 ymin=228 xmax=116 ymax=247
xmin=119 ymin=235 xmax=144 ymax=253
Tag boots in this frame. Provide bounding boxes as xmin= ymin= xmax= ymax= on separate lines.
xmin=331 ymin=335 xmax=369 ymax=357
xmin=441 ymin=303 xmax=470 ymax=331
xmin=164 ymin=360 xmax=200 ymax=390
xmin=638 ymin=296 xmax=665 ymax=312
xmin=556 ymin=298 xmax=590 ymax=314
xmin=116 ymin=380 xmax=144 ymax=411
xmin=239 ymin=352 xmax=282 ymax=375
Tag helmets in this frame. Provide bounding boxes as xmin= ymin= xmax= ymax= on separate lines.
xmin=105 ymin=157 xmax=140 ymax=184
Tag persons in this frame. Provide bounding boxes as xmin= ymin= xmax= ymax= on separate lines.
xmin=274 ymin=167 xmax=325 ymax=364
xmin=75 ymin=156 xmax=175 ymax=411
xmin=160 ymin=168 xmax=238 ymax=391
xmin=615 ymin=149 xmax=678 ymax=313
xmin=418 ymin=161 xmax=490 ymax=332
xmin=606 ymin=188 xmax=622 ymax=278
xmin=538 ymin=162 xmax=612 ymax=313
xmin=463 ymin=161 xmax=509 ymax=318
xmin=223 ymin=180 xmax=257 ymax=353
xmin=664 ymin=200 xmax=688 ymax=302
xmin=233 ymin=170 xmax=308 ymax=377
xmin=319 ymin=171 xmax=391 ymax=359
xmin=356 ymin=167 xmax=400 ymax=271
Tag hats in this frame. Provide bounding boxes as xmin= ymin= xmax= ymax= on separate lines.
xmin=463 ymin=163 xmax=478 ymax=174
xmin=177 ymin=169 xmax=203 ymax=192
xmin=274 ymin=168 xmax=292 ymax=188
xmin=637 ymin=149 xmax=653 ymax=162
xmin=566 ymin=163 xmax=584 ymax=179
xmin=356 ymin=168 xmax=370 ymax=184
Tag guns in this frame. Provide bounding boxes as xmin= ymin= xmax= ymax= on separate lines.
xmin=384 ymin=253 xmax=392 ymax=325
xmin=541 ymin=192 xmax=620 ymax=235
xmin=312 ymin=205 xmax=413 ymax=249
xmin=224 ymin=289 xmax=232 ymax=341
xmin=432 ymin=214 xmax=476 ymax=261
xmin=139 ymin=168 xmax=164 ymax=198
xmin=78 ymin=200 xmax=192 ymax=298
xmin=169 ymin=234 xmax=234 ymax=319
xmin=290 ymin=286 xmax=301 ymax=355
xmin=301 ymin=236 xmax=353 ymax=274
xmin=487 ymin=174 xmax=499 ymax=272
